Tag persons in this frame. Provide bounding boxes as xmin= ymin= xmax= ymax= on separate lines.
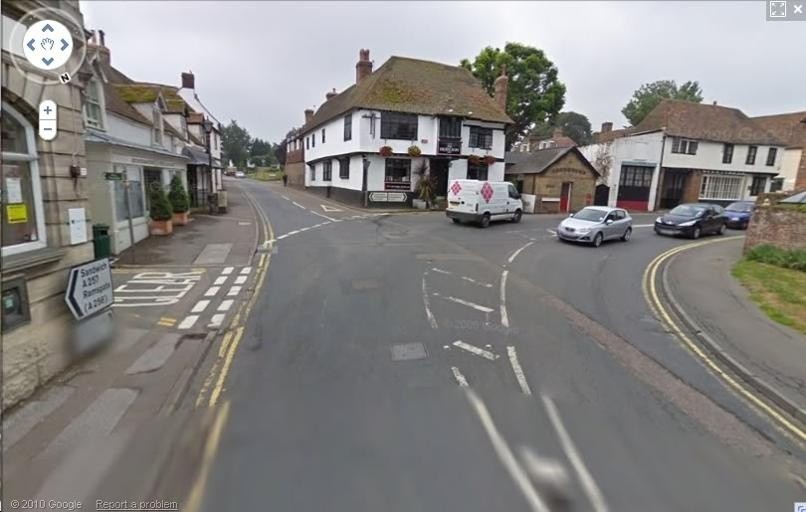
xmin=282 ymin=172 xmax=288 ymax=187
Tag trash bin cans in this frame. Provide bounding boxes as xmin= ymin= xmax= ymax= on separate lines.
xmin=208 ymin=189 xmax=227 ymax=214
xmin=92 ymin=223 xmax=110 ymax=259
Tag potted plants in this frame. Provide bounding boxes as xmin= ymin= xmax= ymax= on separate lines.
xmin=148 ymin=174 xmax=190 ymax=236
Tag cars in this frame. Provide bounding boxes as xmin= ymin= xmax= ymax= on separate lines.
xmin=234 ymin=171 xmax=247 ymax=178
xmin=556 ymin=201 xmax=756 ymax=248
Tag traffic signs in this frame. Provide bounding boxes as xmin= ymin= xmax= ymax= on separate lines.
xmin=63 ymin=256 xmax=114 ymax=322
xmin=369 ymin=193 xmax=407 ymax=203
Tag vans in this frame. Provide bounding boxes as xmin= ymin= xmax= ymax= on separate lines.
xmin=445 ymin=180 xmax=524 ymax=228
